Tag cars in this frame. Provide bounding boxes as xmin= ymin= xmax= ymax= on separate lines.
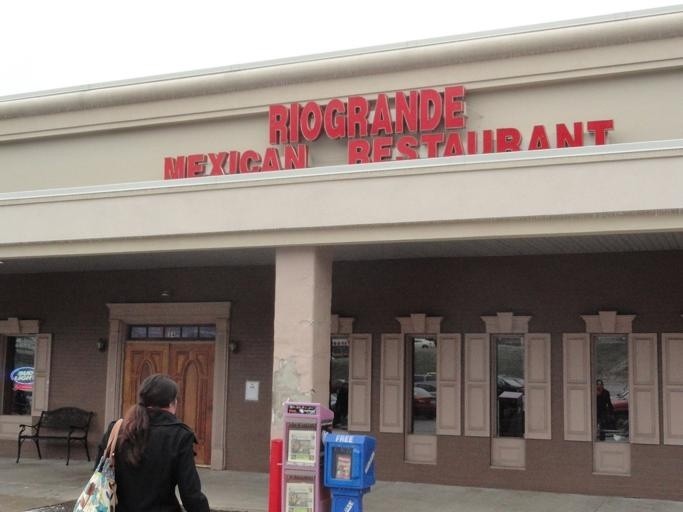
xmin=415 ymin=339 xmax=436 ymax=350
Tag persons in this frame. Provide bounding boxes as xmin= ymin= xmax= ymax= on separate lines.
xmin=93 ymin=373 xmax=211 ymax=511
xmin=594 ymin=378 xmax=618 ymax=440
xmin=9 ymin=378 xmax=32 ymax=415
xmin=331 ymin=382 xmax=347 ymax=430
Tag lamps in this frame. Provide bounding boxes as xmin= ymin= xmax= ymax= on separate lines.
xmin=94 ymin=337 xmax=107 ymax=352
xmin=228 ymin=339 xmax=238 ymax=354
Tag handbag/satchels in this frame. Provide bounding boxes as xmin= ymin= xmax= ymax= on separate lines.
xmin=72 ymin=416 xmax=123 ymax=512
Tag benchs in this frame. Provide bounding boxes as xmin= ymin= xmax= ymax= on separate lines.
xmin=16 ymin=406 xmax=95 ymax=466
